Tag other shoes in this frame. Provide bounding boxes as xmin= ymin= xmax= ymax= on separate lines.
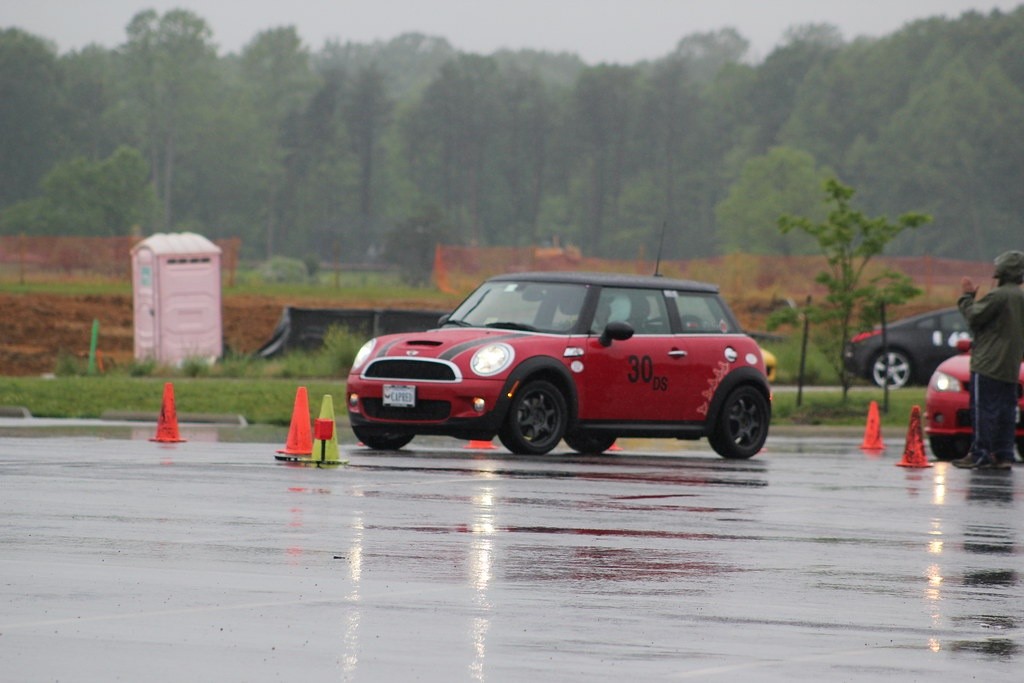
xmin=953 ymin=451 xmax=990 ymax=468
xmin=987 ymin=454 xmax=1012 ymax=468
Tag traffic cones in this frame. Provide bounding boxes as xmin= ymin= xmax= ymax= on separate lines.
xmin=355 ymin=442 xmax=365 ymax=446
xmin=299 ymin=394 xmax=349 ymax=469
xmin=606 ymin=439 xmax=625 ymax=452
xmin=894 ymin=405 xmax=936 ymax=467
xmin=858 ymin=399 xmax=888 ymax=450
xmin=274 ymin=386 xmax=313 ymax=457
xmin=463 ymin=437 xmax=499 ymax=452
xmin=149 ymin=383 xmax=187 ymax=443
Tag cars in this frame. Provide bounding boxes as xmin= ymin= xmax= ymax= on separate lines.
xmin=843 ymin=305 xmax=976 ymax=390
xmin=923 ymin=339 xmax=1024 ymax=461
xmin=344 ymin=270 xmax=777 ymax=456
xmin=758 ymin=346 xmax=776 ymax=383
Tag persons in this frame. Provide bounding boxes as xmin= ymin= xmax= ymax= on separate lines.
xmin=952 ymin=250 xmax=1024 ymax=468
xmin=596 ymin=293 xmax=632 ymax=326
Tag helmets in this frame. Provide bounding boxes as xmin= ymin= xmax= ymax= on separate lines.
xmin=591 ymin=291 xmax=632 ymax=330
